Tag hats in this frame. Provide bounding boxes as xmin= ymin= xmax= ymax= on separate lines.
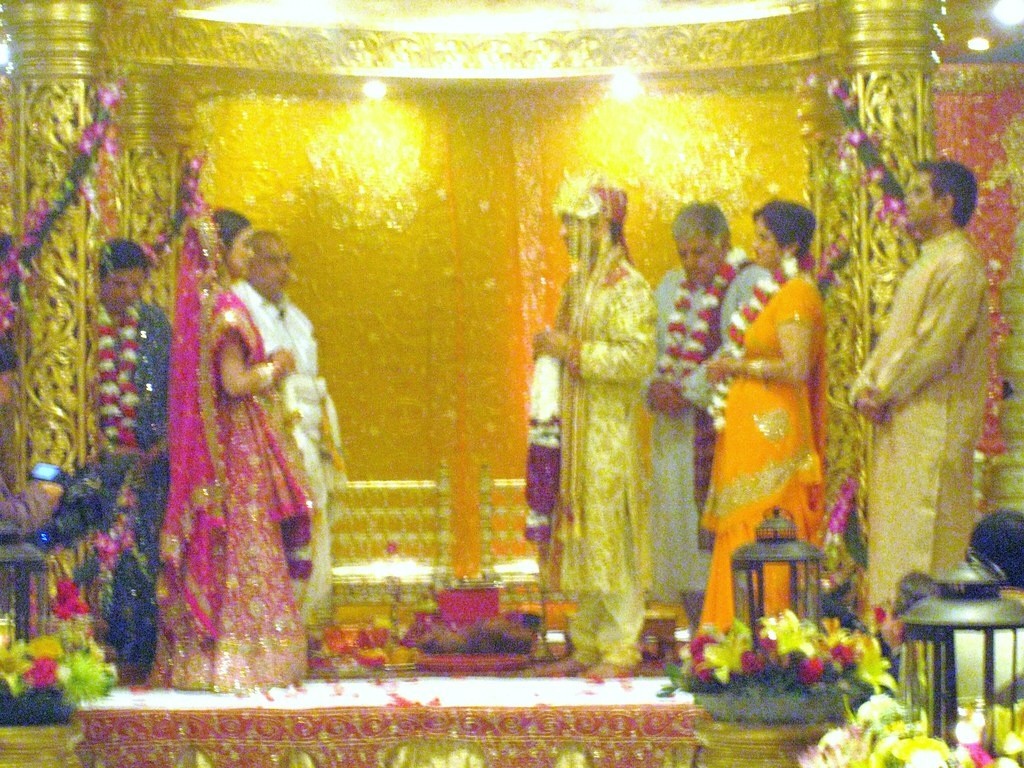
xmin=559 ymin=173 xmax=627 ymax=223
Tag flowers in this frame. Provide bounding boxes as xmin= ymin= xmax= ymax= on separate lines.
xmin=659 ymin=247 xmax=747 ymax=379
xmin=816 ymin=234 xmax=849 ymax=294
xmin=828 ymin=76 xmax=927 ymax=246
xmin=728 ymin=250 xmax=817 ymax=350
xmin=667 ymin=606 xmax=902 ymax=694
xmin=0 ymin=626 xmax=117 ymax=724
xmin=1 ymin=81 xmax=123 ymax=333
xmin=138 ymin=152 xmax=209 ymax=265
xmin=401 ymin=608 xmax=544 ymax=654
xmin=93 ymin=300 xmax=140 ymax=452
xmin=795 ymin=694 xmax=1024 ymax=768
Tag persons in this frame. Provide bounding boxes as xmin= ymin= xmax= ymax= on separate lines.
xmin=842 ymin=159 xmax=991 ymax=646
xmin=0 ymin=339 xmax=64 ymax=629
xmin=697 ymin=202 xmax=833 ymax=682
xmin=81 ymin=237 xmax=176 ymax=680
xmin=226 ymin=228 xmax=343 ymax=627
xmin=647 ymin=198 xmax=743 ymax=678
xmin=188 ymin=207 xmax=308 ymax=690
xmin=525 ymin=172 xmax=665 ymax=684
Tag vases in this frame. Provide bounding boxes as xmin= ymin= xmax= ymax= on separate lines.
xmin=0 ymin=714 xmax=86 ymax=768
xmin=696 ymin=695 xmax=854 ymax=725
xmin=416 ymin=654 xmax=528 ymax=675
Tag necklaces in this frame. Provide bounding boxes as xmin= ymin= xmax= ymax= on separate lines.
xmin=661 ymin=251 xmax=742 ymax=382
xmin=708 ymin=257 xmax=810 ymax=432
